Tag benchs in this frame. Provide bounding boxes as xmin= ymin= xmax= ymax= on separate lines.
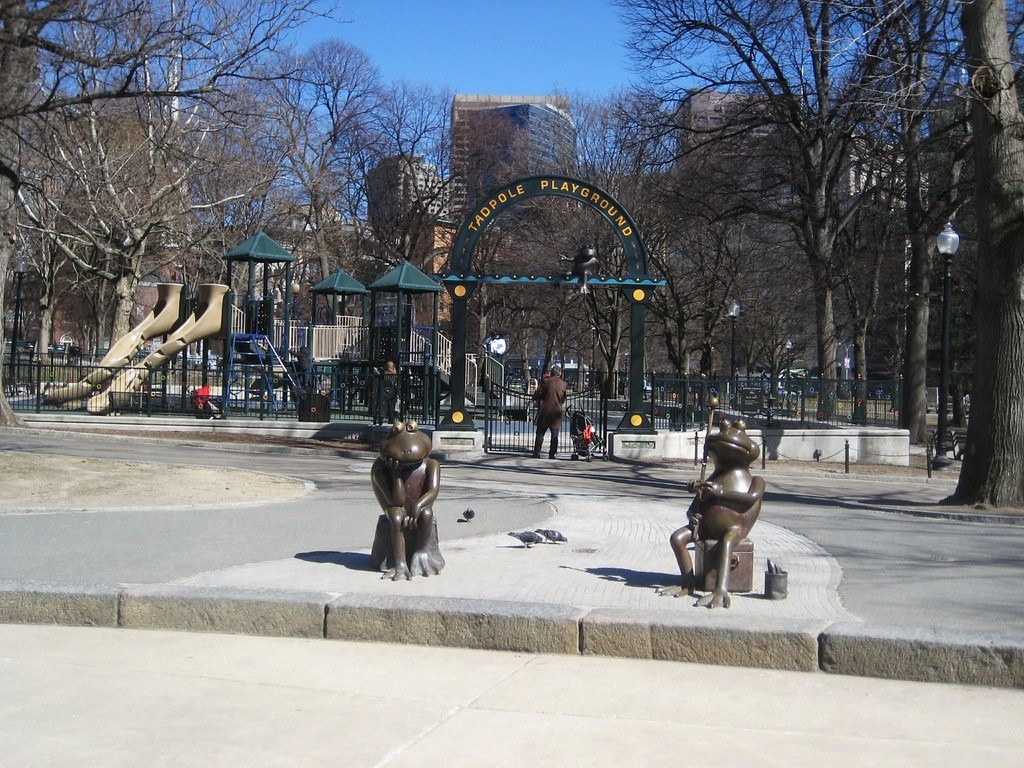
xmin=761 ymin=408 xmax=797 ymax=419
xmin=933 ymin=430 xmax=967 ymax=460
xmin=3 ymin=372 xmax=21 ymax=396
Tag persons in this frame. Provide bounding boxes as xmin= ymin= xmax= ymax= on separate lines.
xmin=534 ymin=373 xmax=550 ymax=426
xmin=370 ymin=360 xmax=400 ymax=424
xmin=290 ymin=347 xmax=311 ymax=388
xmin=532 ymin=366 xmax=568 ymax=459
xmin=643 ymin=378 xmax=648 ymax=401
xmin=485 ymin=329 xmax=509 ymax=365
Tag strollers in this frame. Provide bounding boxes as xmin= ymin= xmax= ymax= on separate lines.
xmin=565 ymin=404 xmax=609 ymax=463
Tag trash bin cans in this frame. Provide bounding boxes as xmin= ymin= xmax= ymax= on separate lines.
xmin=299 ymin=388 xmax=332 ymax=423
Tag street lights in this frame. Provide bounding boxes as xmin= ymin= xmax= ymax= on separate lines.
xmin=728 ymin=299 xmax=739 ymax=404
xmin=786 ymin=339 xmax=792 ymax=417
xmin=838 ymin=342 xmax=854 ymax=392
xmin=932 ymin=221 xmax=960 ymax=473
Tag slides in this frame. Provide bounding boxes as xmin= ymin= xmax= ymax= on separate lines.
xmin=43 ymin=283 xmax=188 ymax=410
xmin=86 ymin=283 xmax=230 ymax=416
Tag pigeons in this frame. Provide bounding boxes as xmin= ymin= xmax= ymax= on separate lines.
xmin=534 ymin=529 xmax=567 ymax=549
xmin=462 ymin=508 xmax=475 ymax=522
xmin=507 ymin=531 xmax=547 ymax=549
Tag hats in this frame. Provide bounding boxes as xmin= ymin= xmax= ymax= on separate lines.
xmin=551 ymin=365 xmax=562 ymax=375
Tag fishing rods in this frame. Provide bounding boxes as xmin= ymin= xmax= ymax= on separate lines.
xmin=686 ymin=396 xmax=719 ymax=543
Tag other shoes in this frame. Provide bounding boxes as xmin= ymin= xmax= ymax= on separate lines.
xmin=549 ymin=452 xmax=556 ymax=458
xmin=532 ymin=452 xmax=540 ymax=458
